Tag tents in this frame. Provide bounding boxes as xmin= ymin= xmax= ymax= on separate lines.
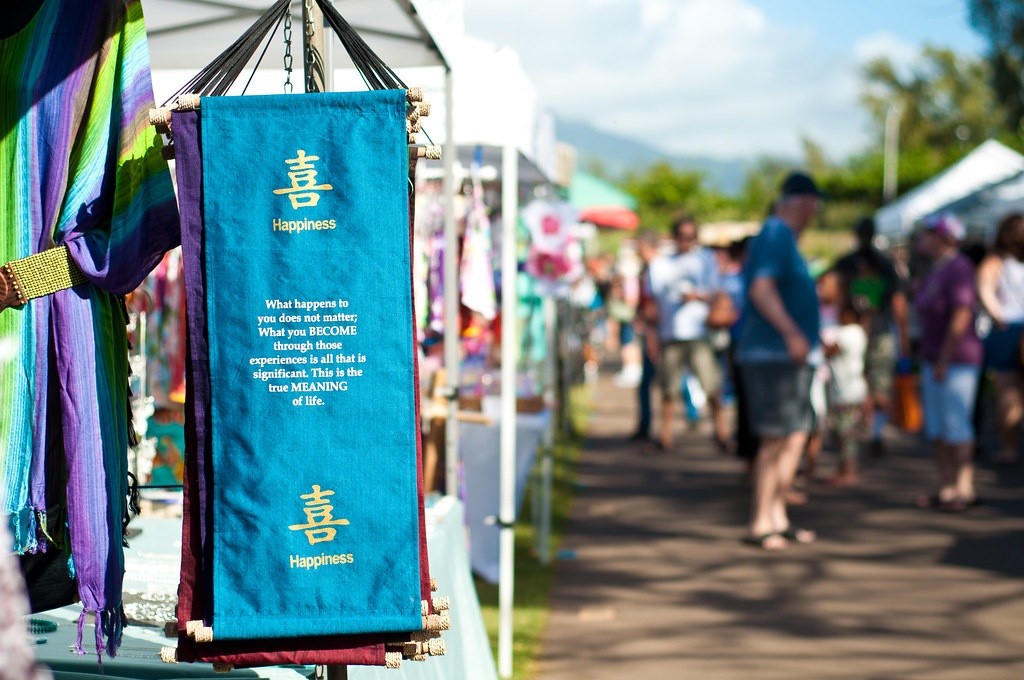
xmin=872 ymin=139 xmax=1024 ymax=255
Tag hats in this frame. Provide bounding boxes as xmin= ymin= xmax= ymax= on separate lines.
xmin=777 ymin=171 xmax=830 ymax=199
xmin=923 ymin=213 xmax=965 ymax=245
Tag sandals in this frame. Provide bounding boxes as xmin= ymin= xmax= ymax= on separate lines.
xmin=740 ymin=526 xmax=807 ymax=550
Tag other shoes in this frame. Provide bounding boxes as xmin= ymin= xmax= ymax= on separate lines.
xmin=917 ymin=490 xmax=983 ymax=512
xmin=628 ymin=424 xmax=866 ymax=504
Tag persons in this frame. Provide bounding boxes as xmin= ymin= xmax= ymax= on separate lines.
xmin=806 ymin=209 xmax=1024 ymax=512
xmin=627 ymin=223 xmax=701 ymax=448
xmin=647 ymin=213 xmax=737 ymax=452
xmin=610 ymin=240 xmax=654 ymax=388
xmin=736 ymin=171 xmax=833 ymax=554
xmin=580 ymin=249 xmax=623 ymax=387
xmin=707 ymin=234 xmax=760 ymax=455
